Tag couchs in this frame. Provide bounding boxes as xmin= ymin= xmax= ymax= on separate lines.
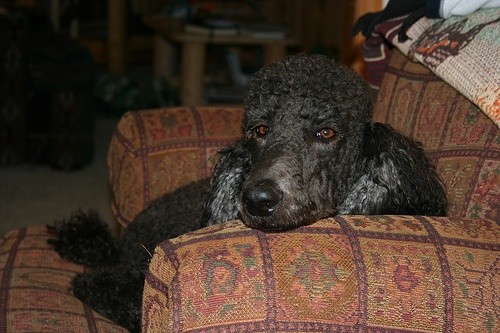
xmin=0 ymin=46 xmax=500 ymax=333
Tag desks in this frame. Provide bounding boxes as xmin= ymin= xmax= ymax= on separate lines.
xmin=142 ymin=13 xmax=287 ymax=107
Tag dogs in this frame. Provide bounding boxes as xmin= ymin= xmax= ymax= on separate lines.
xmin=43 ymin=50 xmax=451 ymax=333
